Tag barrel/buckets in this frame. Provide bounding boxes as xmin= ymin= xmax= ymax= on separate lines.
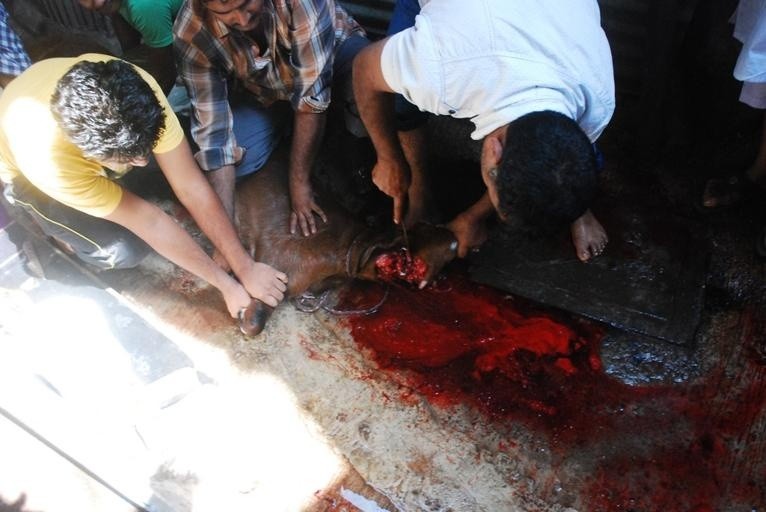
xmin=693 ymin=169 xmax=758 ymax=216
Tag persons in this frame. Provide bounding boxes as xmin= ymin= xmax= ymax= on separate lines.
xmin=172 ymin=0 xmax=371 ymax=275
xmin=698 ymin=0 xmax=766 ymax=257
xmin=79 ymin=0 xmax=182 ymax=65
xmin=0 ymin=1 xmax=33 ymax=89
xmin=0 ymin=53 xmax=289 ymax=318
xmin=351 ymin=0 xmax=617 ymax=262
